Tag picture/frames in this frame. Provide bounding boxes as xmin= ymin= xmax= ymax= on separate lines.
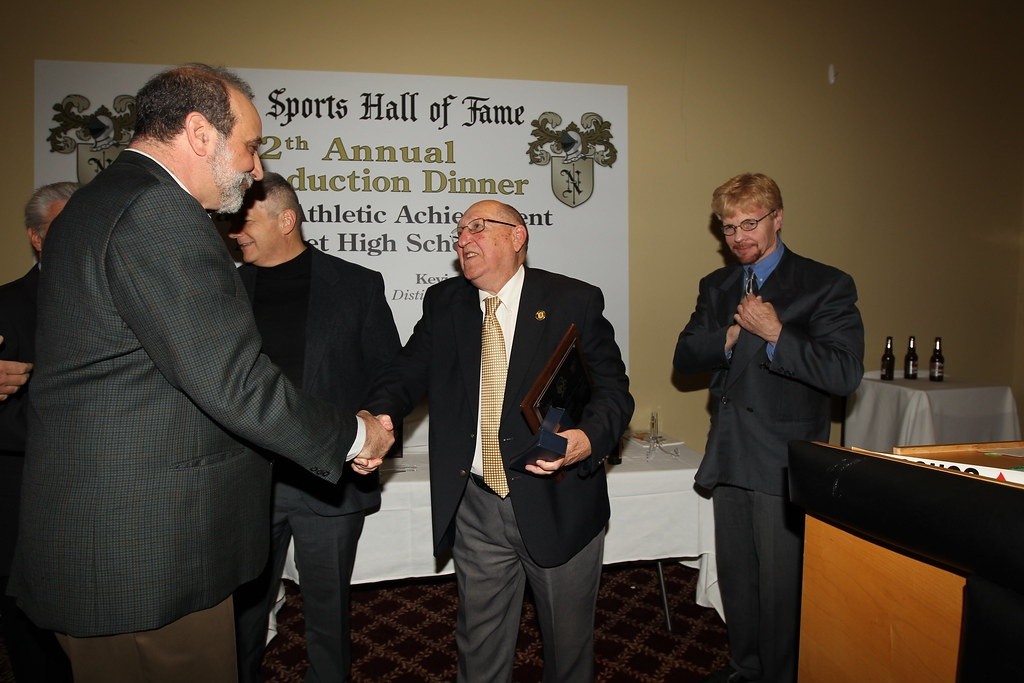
xmin=519 ymin=322 xmax=583 ymax=435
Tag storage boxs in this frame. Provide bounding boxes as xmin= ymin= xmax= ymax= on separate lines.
xmin=508 ymin=429 xmax=568 ymax=472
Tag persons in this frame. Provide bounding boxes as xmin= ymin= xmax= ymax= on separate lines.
xmin=7 ymin=61 xmax=395 ymax=683
xmin=0 ymin=181 xmax=85 ymax=683
xmin=351 ymin=200 xmax=635 ymax=683
xmin=227 ymin=173 xmax=403 ymax=683
xmin=673 ymin=173 xmax=865 ymax=683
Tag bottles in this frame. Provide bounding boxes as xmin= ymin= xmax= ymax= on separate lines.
xmin=904 ymin=336 xmax=918 ymax=380
xmin=881 ymin=336 xmax=895 ymax=381
xmin=930 ymin=336 xmax=943 ymax=382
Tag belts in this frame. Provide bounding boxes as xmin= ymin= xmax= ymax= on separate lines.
xmin=469 ymin=472 xmax=498 ymax=495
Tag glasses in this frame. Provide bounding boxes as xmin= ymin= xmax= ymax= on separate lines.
xmin=449 ymin=218 xmax=517 ymax=244
xmin=719 ymin=209 xmax=778 ymax=236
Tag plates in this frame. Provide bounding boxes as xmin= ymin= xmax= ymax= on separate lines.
xmin=622 ymin=431 xmax=685 ymax=448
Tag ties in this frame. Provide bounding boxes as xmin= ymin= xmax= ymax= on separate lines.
xmin=744 ymin=268 xmax=759 ymax=297
xmin=481 ymin=297 xmax=510 ymax=500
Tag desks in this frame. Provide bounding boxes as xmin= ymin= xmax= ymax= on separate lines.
xmin=844 ymin=370 xmax=1022 ymax=454
xmin=266 ymin=432 xmax=727 ymax=648
